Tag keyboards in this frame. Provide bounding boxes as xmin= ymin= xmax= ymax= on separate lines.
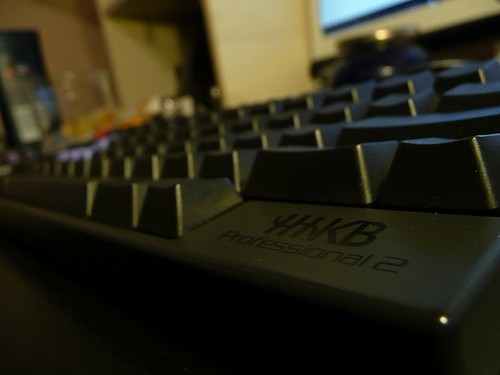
xmin=0 ymin=56 xmax=500 ymax=375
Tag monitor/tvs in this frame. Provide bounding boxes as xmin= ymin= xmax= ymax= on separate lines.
xmin=304 ymin=0 xmax=499 ymax=60
